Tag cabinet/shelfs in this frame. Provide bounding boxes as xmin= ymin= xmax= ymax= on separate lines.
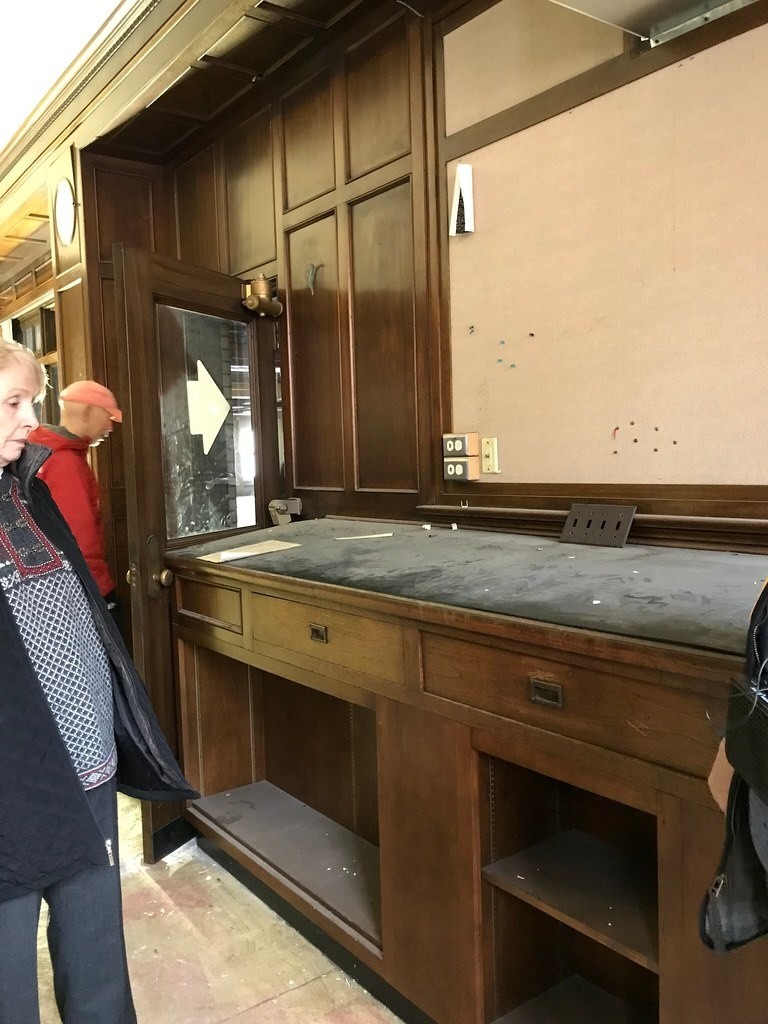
xmin=165 ymin=516 xmax=767 ymax=1023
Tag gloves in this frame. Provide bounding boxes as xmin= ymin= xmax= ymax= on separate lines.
xmin=104 ymin=590 xmax=118 ymax=611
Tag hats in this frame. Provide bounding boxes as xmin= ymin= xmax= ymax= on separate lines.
xmin=59 ymin=381 xmax=123 ymax=424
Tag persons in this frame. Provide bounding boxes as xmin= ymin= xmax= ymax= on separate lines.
xmin=0 ymin=338 xmax=201 ymax=1024
xmin=699 ymin=582 xmax=768 ymax=953
xmin=28 ymin=380 xmax=123 ymax=612
